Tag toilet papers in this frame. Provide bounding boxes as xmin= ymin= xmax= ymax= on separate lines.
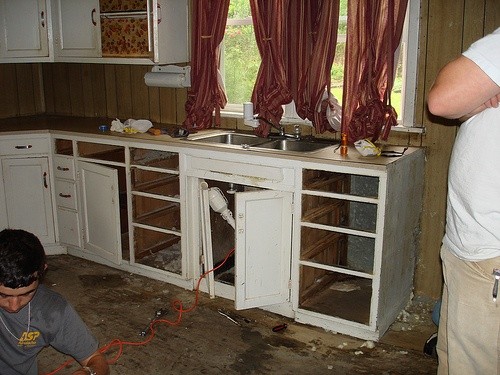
xmin=144 ymin=72 xmax=185 ymax=88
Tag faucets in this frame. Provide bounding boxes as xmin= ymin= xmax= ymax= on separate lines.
xmin=249 ymin=117 xmax=302 ymax=141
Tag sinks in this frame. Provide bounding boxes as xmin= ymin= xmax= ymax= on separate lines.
xmin=184 ymin=131 xmax=276 ymax=148
xmin=249 ymin=137 xmax=335 ymax=153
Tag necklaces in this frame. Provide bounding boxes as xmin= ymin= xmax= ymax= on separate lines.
xmin=0 ymin=302 xmax=30 ymax=341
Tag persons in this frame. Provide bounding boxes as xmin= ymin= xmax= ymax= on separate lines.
xmin=428 ymin=26 xmax=500 ymax=375
xmin=0 ymin=228 xmax=110 ymax=375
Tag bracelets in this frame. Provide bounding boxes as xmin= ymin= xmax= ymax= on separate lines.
xmin=82 ymin=365 xmax=96 ymax=375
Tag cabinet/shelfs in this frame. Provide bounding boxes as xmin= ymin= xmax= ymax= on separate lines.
xmin=74 ymin=140 xmax=187 ymax=290
xmin=0 ymin=0 xmax=190 ymax=65
xmin=0 ymin=137 xmax=55 ymax=246
xmin=289 ymin=140 xmax=427 ymax=339
xmin=50 ymin=136 xmax=83 ymax=253
xmin=184 ymin=156 xmax=294 ymax=320
